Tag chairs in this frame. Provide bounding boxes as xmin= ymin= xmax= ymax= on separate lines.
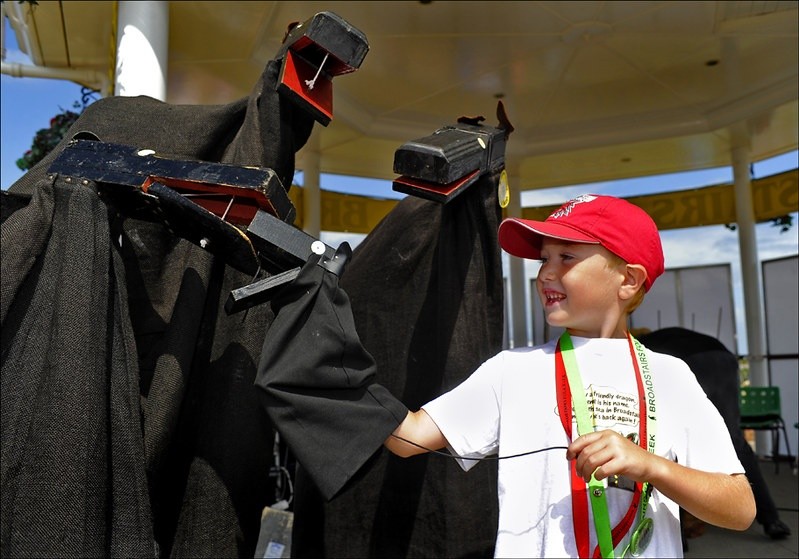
xmin=738 ymin=386 xmax=794 ymax=475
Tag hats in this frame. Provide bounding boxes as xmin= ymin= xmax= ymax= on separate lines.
xmin=498 ymin=193 xmax=665 ymax=293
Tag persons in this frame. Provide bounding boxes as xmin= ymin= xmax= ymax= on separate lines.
xmin=385 ymin=194 xmax=756 ymax=559
